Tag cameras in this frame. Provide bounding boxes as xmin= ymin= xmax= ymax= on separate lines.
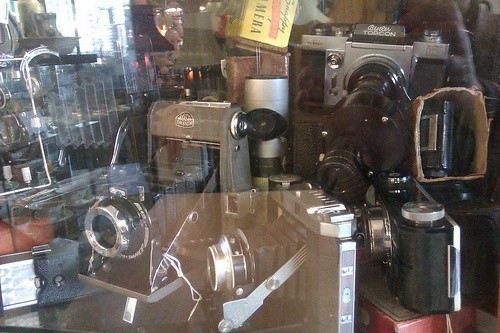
xmin=1 ymin=19 xmax=499 ymax=333
